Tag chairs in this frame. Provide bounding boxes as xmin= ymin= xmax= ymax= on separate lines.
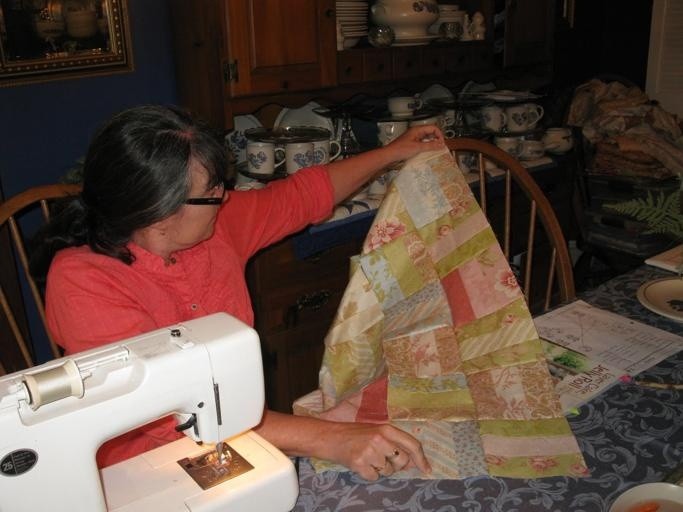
xmin=1 ymin=184 xmax=85 ymax=377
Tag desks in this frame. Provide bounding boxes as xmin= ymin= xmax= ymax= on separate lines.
xmin=290 ymin=265 xmax=682 ymax=512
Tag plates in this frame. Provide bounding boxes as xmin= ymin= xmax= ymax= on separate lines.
xmin=336 ymin=2 xmax=374 ymax=51
xmin=635 ymin=276 xmax=683 ymax=326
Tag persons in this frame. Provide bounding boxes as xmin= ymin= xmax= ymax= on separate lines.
xmin=24 ymin=103 xmax=445 ymax=483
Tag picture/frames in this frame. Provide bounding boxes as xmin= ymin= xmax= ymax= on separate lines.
xmin=0 ymin=0 xmax=136 ymax=89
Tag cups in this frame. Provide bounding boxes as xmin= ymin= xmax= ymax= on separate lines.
xmin=247 ymin=141 xmax=341 ymax=174
xmin=378 ymin=84 xmax=576 ymax=161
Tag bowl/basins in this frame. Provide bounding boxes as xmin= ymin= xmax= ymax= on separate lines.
xmin=609 ymin=482 xmax=683 ymax=509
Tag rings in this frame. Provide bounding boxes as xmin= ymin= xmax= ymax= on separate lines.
xmin=388 ymin=446 xmax=401 ymax=461
xmin=374 ymin=459 xmax=388 ymax=471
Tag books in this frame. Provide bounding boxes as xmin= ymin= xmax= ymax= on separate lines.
xmin=532 ymin=300 xmax=683 ymax=416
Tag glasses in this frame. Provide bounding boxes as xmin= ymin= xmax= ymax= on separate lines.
xmin=181 ymin=177 xmax=227 ymax=205
